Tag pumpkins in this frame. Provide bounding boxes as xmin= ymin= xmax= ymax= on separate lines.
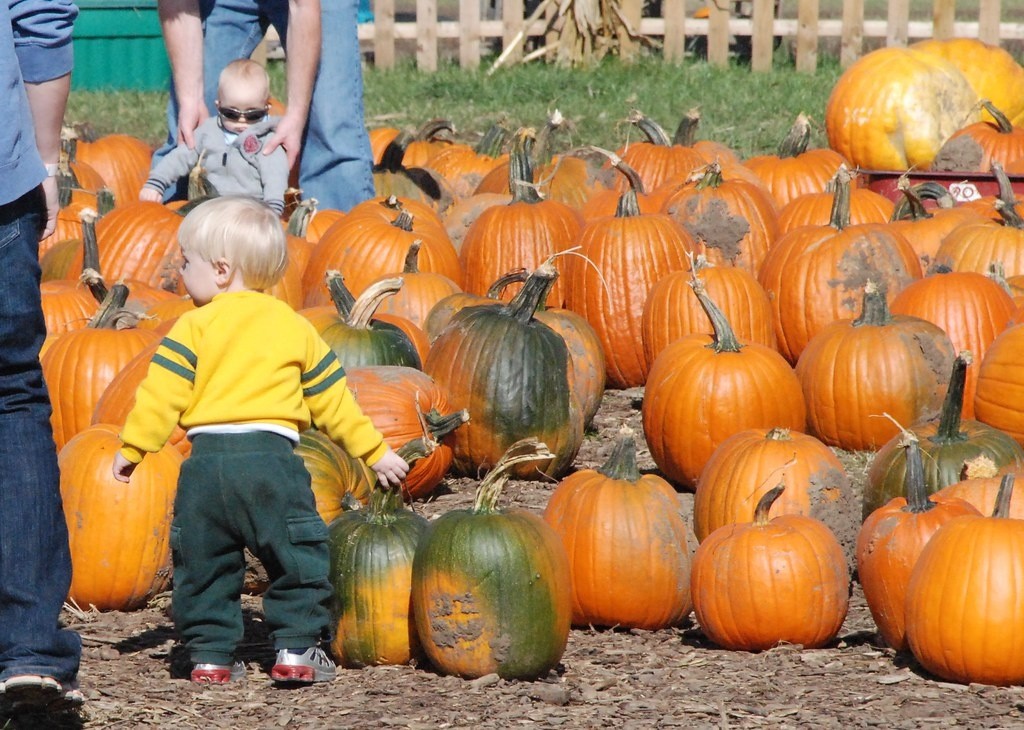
xmin=40 ymin=34 xmax=1024 ymax=685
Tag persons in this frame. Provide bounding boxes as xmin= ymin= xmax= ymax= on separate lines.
xmin=112 ymin=196 xmax=408 ymax=684
xmin=138 ymin=59 xmax=289 ymax=217
xmin=0 ymin=0 xmax=89 ymax=714
xmin=152 ymin=0 xmax=376 ymax=215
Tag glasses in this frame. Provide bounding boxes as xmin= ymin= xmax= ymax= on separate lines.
xmin=219 ymin=105 xmax=269 ymax=121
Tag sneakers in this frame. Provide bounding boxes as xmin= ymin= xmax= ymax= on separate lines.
xmin=191 ymin=661 xmax=247 ymax=684
xmin=271 ymin=647 xmax=336 ymax=682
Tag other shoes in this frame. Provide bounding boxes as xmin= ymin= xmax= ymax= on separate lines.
xmin=0 ymin=675 xmax=84 ymax=730
